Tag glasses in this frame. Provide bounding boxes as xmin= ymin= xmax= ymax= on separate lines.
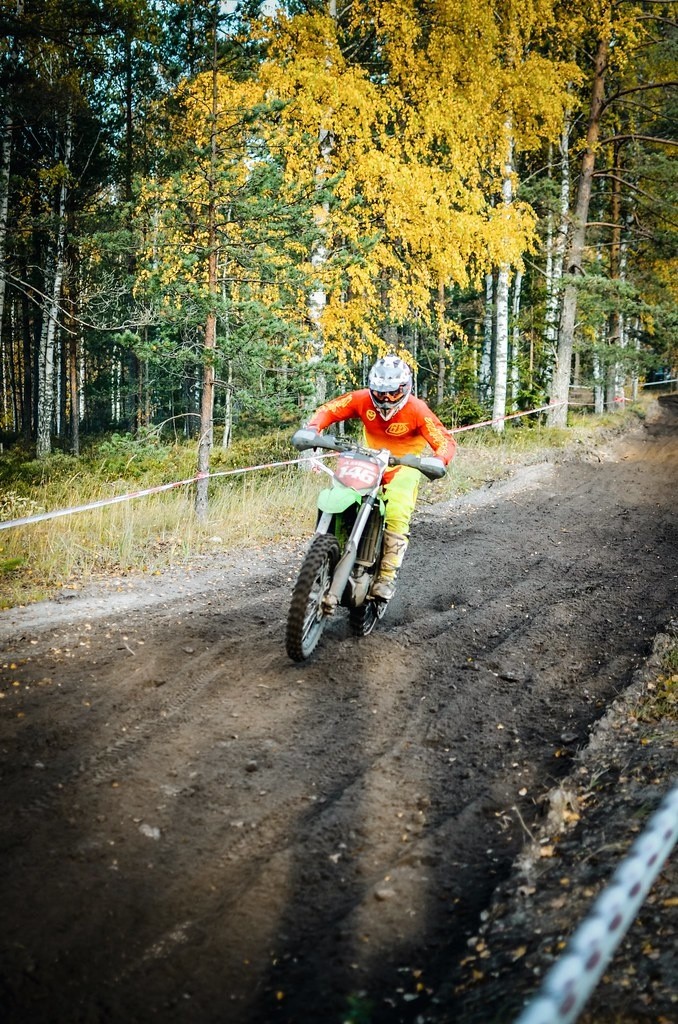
xmin=371 ymin=386 xmax=405 ymax=403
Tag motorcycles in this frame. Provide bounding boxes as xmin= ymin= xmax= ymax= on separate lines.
xmin=286 ymin=429 xmax=447 ymax=666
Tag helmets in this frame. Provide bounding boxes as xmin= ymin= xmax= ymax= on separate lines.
xmin=368 ymin=357 xmax=412 ymax=421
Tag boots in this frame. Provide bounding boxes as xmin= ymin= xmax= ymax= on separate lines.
xmin=371 ymin=529 xmax=409 ymax=599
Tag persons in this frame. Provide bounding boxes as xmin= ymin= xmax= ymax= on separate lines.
xmin=303 ymin=358 xmax=456 ymax=601
